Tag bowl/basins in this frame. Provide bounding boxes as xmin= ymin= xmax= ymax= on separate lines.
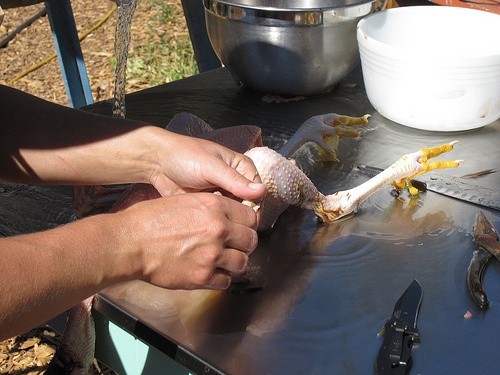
xmin=356 ymin=5 xmax=500 ymax=134
xmin=202 ymin=0 xmax=375 ymax=97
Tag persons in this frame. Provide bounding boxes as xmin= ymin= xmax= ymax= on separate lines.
xmin=0 ymin=84 xmax=265 ymax=343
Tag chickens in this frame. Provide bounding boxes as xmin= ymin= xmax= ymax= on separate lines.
xmin=72 ymin=112 xmax=464 ymax=282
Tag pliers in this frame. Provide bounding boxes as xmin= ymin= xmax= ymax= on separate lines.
xmin=467 ymin=211 xmax=500 ymax=311
